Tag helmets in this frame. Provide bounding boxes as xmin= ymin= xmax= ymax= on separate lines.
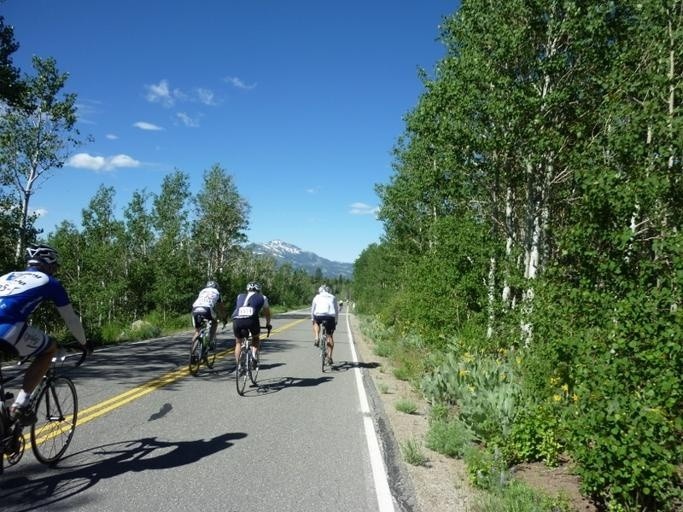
xmin=24 ymin=243 xmax=58 ymax=266
xmin=247 ymin=281 xmax=262 ymax=291
xmin=319 ymin=284 xmax=331 ymax=293
xmin=207 ymin=281 xmax=218 ymax=288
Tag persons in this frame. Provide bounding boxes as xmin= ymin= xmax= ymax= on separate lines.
xmin=190 ymin=280 xmax=228 ymax=355
xmin=311 ymin=284 xmax=339 ymax=364
xmin=231 ymin=281 xmax=271 ymax=377
xmin=0 ymin=244 xmax=94 ymax=426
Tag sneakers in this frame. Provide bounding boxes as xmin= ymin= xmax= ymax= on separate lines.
xmin=251 ymin=357 xmax=257 ymax=368
xmin=206 ymin=343 xmax=215 ymax=351
xmin=327 ymin=358 xmax=333 ymax=364
xmin=10 ymin=403 xmax=37 ymax=425
xmin=239 ymin=368 xmax=245 ymax=376
xmin=315 ymin=338 xmax=319 ymax=345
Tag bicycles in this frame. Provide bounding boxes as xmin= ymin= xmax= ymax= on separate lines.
xmin=0 ymin=341 xmax=87 ymax=470
xmin=189 ymin=315 xmax=227 ymax=376
xmin=236 ymin=326 xmax=272 ymax=395
xmin=315 ymin=321 xmax=329 ymax=373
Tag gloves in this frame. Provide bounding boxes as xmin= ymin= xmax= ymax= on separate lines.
xmin=85 ymin=338 xmax=94 ymax=351
